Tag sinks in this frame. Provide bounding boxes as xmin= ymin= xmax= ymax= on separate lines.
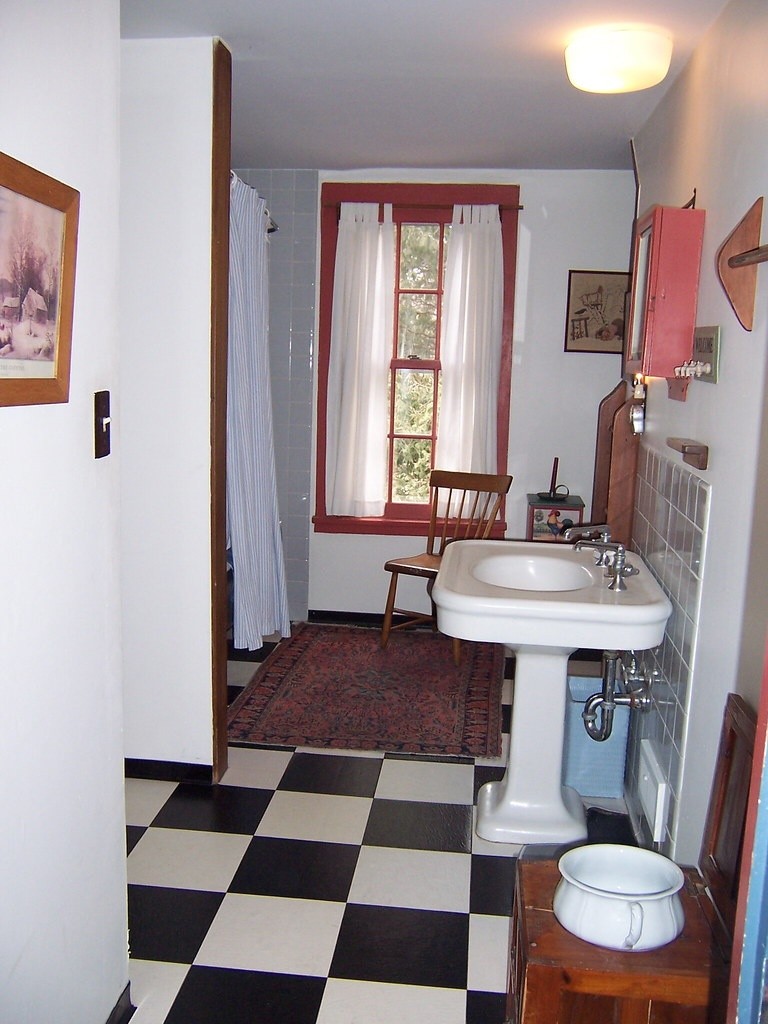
xmin=431 ymin=539 xmax=674 ymax=651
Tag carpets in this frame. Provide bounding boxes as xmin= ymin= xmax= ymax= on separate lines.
xmin=228 ymin=623 xmax=504 ymax=758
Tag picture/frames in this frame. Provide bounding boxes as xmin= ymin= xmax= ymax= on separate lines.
xmin=0 ymin=148 xmax=81 ymax=406
xmin=564 ymin=269 xmax=628 ymax=354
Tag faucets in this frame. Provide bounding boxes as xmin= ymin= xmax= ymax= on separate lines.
xmin=572 ymin=540 xmax=627 ymax=592
xmin=563 ymin=525 xmax=611 ymax=542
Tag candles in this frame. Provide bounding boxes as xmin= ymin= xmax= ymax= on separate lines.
xmin=636 ymin=374 xmax=643 ymax=385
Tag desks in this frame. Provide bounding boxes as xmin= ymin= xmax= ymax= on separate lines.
xmin=526 ymin=494 xmax=586 ymax=544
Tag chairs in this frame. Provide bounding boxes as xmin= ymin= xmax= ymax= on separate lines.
xmin=380 ymin=470 xmax=513 ymax=667
xmin=512 ymin=694 xmax=757 ymax=1024
